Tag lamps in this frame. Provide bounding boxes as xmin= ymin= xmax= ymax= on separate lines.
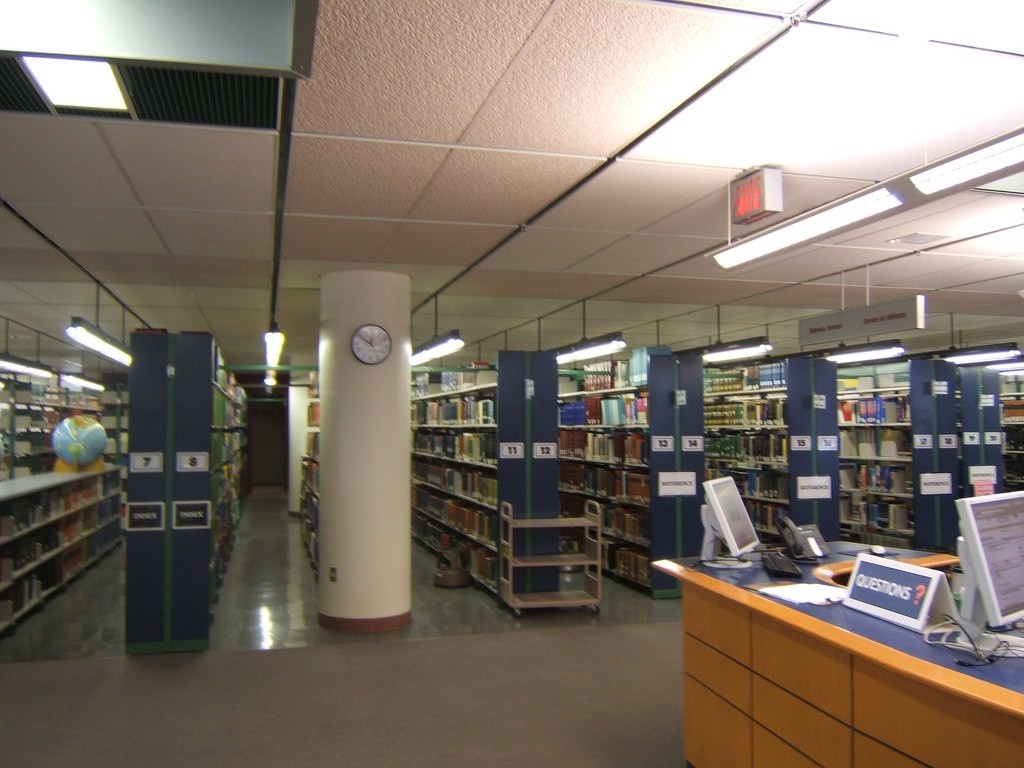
xmin=552 ymin=325 xmax=627 ymax=366
xmin=67 ymin=315 xmax=132 ymax=367
xmin=711 ymin=123 xmax=1022 ymax=271
xmin=700 ymin=308 xmax=775 ymax=365
xmin=260 ymin=324 xmax=285 ymax=387
xmin=0 ymin=354 xmax=55 ymax=379
xmin=824 ymin=336 xmax=903 ymax=364
xmin=409 ymin=328 xmax=466 ymax=371
xmin=62 ymin=373 xmax=111 ymax=393
xmin=937 ymin=343 xmax=1023 ymax=380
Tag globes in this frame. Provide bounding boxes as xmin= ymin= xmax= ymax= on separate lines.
xmin=52 ymin=414 xmax=109 ymax=472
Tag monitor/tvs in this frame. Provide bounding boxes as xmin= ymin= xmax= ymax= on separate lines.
xmin=697 ymin=475 xmax=760 ymax=569
xmin=953 ymin=491 xmax=1024 ymax=657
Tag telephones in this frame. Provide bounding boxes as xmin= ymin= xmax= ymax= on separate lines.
xmin=774 ymin=515 xmax=830 ymax=561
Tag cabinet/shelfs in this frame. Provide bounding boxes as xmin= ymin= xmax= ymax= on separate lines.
xmin=710 ymin=354 xmax=838 ymax=556
xmin=405 ymin=350 xmax=565 ymax=595
xmin=559 ymin=339 xmax=706 ymax=598
xmin=838 ymin=362 xmax=1024 ymax=552
xmin=498 ymin=501 xmax=606 ymax=615
xmin=294 ymin=370 xmax=326 ymax=576
xmin=0 ymin=327 xmax=249 ymax=657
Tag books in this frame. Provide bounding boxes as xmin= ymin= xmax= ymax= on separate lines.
xmin=838 ymin=462 xmax=906 ymax=493
xmin=559 ymin=392 xmax=649 ymax=465
xmin=744 ymin=499 xmax=790 ymax=530
xmin=704 ymin=430 xmax=788 ymax=463
xmin=705 ymin=467 xmax=789 ymax=499
xmin=412 ymin=460 xmax=498 ymax=507
xmin=703 ymin=360 xmax=789 ymax=392
xmin=411 ymin=395 xmax=497 ymax=462
xmin=0 ymin=379 xmax=103 ymax=481
xmin=559 ymin=462 xmax=651 ymax=585
xmin=837 ymin=396 xmax=915 ymax=424
xmin=213 ymin=347 xmax=249 ymax=551
xmin=414 ymin=486 xmax=498 ymax=592
xmin=838 ymin=494 xmax=913 ymax=550
xmin=839 ymin=427 xmax=901 ymax=457
xmin=703 ymin=398 xmax=787 ymax=426
xmin=0 ymin=474 xmax=116 ymax=620
xmin=1000 ymin=399 xmax=1024 ymax=492
xmin=558 ymin=346 xmax=650 ymax=388
xmin=299 ymin=373 xmax=319 ymax=566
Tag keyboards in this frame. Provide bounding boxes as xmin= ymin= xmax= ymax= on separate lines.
xmin=762 ymin=552 xmax=803 ymax=578
xmin=869 ymin=545 xmax=886 ymax=554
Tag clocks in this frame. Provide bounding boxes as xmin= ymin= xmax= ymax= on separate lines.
xmin=350 ymin=325 xmax=393 ymax=366
